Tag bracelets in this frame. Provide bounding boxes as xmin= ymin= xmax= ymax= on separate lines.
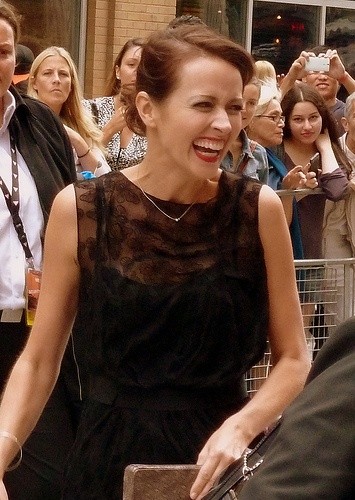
xmin=0 ymin=432 xmax=22 ymax=472
xmin=77 ymin=145 xmax=90 ymax=158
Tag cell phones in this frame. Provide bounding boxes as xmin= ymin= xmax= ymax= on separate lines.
xmin=304 ymin=57 xmax=330 ymax=72
xmin=309 ymin=152 xmax=320 ymax=181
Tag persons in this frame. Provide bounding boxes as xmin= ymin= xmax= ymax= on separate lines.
xmin=203 ymin=316 xmax=355 ymax=500
xmin=0 ymin=0 xmax=355 ymax=397
xmin=0 ymin=20 xmax=313 ymax=500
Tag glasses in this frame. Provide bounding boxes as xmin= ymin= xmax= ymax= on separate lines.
xmin=254 ymin=115 xmax=285 ymax=123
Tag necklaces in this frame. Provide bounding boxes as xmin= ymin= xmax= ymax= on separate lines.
xmin=136 ymin=164 xmax=207 ymax=221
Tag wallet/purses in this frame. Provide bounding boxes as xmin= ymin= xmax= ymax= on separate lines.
xmin=122 ymin=464 xmax=201 ymax=500
